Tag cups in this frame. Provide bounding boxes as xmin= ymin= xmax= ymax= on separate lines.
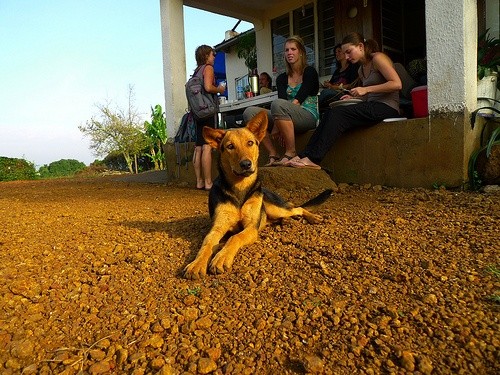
xmin=218 ymin=97 xmax=226 ymax=105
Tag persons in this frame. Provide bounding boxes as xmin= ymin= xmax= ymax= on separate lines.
xmin=190 ymin=45 xmax=226 ymax=190
xmin=283 ymin=33 xmax=402 ymax=168
xmin=256 ymin=72 xmax=276 ymax=96
xmin=243 ymin=35 xmax=319 ymax=166
xmin=318 ymin=43 xmax=360 ymax=115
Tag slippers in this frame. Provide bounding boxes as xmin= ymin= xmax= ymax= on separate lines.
xmin=284 ymin=156 xmax=300 ymax=166
xmin=195 ymin=186 xmax=212 ymax=190
xmin=291 ymin=160 xmax=321 ymax=170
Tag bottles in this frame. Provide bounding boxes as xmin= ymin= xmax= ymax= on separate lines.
xmin=237 ymin=83 xmax=244 ymax=100
xmin=251 ymin=68 xmax=260 ymax=97
xmin=217 ymin=80 xmax=227 ymax=95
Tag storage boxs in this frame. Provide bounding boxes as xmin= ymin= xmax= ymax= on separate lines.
xmin=410 ymin=85 xmax=428 ymax=118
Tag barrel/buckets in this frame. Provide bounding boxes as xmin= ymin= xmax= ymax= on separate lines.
xmin=411 ymin=86 xmax=428 ymax=117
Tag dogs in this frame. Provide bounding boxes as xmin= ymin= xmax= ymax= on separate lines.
xmin=181 ymin=110 xmax=333 ymax=279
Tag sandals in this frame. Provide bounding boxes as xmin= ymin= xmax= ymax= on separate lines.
xmin=272 ymin=154 xmax=293 ymax=166
xmin=265 ymin=156 xmax=279 ymax=167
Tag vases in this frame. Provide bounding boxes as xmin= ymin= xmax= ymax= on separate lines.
xmin=478 ymin=71 xmax=498 ymax=114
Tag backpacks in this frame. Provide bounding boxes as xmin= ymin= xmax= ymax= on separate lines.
xmin=185 ymin=63 xmax=221 ymax=118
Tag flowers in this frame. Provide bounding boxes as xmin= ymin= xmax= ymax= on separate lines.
xmin=476 ymin=27 xmax=500 ymax=90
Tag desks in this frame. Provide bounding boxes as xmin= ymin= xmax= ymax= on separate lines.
xmin=219 ymin=91 xmax=279 ymax=130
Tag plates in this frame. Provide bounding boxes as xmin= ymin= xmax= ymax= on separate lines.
xmin=328 ymin=98 xmax=363 ymax=107
